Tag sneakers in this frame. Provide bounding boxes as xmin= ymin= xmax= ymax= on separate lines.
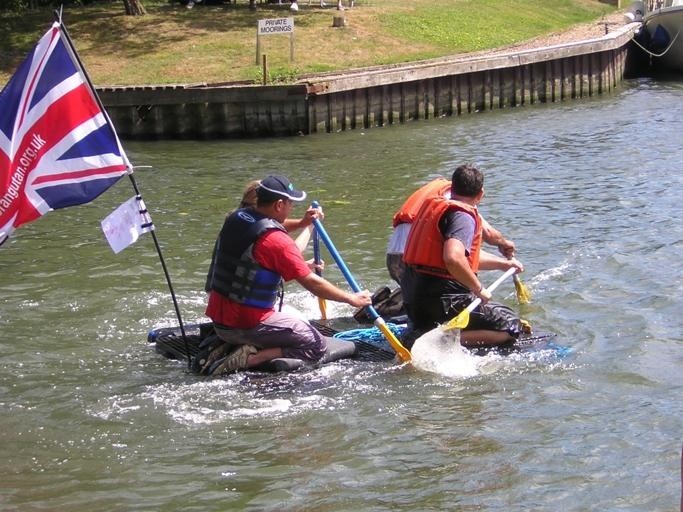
xmin=192 ymin=336 xmax=257 ymax=377
xmin=354 ymin=287 xmax=404 ymax=324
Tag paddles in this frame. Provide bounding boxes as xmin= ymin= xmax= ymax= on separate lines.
xmin=439 ymin=266 xmax=518 ymax=330
xmin=310 ymin=222 xmax=327 ymax=319
xmin=506 ymin=254 xmax=528 ymax=302
xmin=305 ymin=202 xmax=413 ymax=363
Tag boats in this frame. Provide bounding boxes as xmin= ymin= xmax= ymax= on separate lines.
xmin=632 ymin=0 xmax=682 ymax=74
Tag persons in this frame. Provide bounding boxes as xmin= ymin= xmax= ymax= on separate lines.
xmin=353 ymin=178 xmax=516 ymax=321
xmin=193 ymin=174 xmax=374 ymax=377
xmin=401 ymin=163 xmax=522 ymax=347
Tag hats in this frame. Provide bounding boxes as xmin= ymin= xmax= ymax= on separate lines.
xmin=259 ymin=175 xmax=307 ymax=202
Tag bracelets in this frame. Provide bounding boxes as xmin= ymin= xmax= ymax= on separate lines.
xmin=475 ymin=284 xmax=483 ymax=294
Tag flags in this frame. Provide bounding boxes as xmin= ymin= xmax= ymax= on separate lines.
xmin=0 ymin=22 xmax=134 ymax=246
